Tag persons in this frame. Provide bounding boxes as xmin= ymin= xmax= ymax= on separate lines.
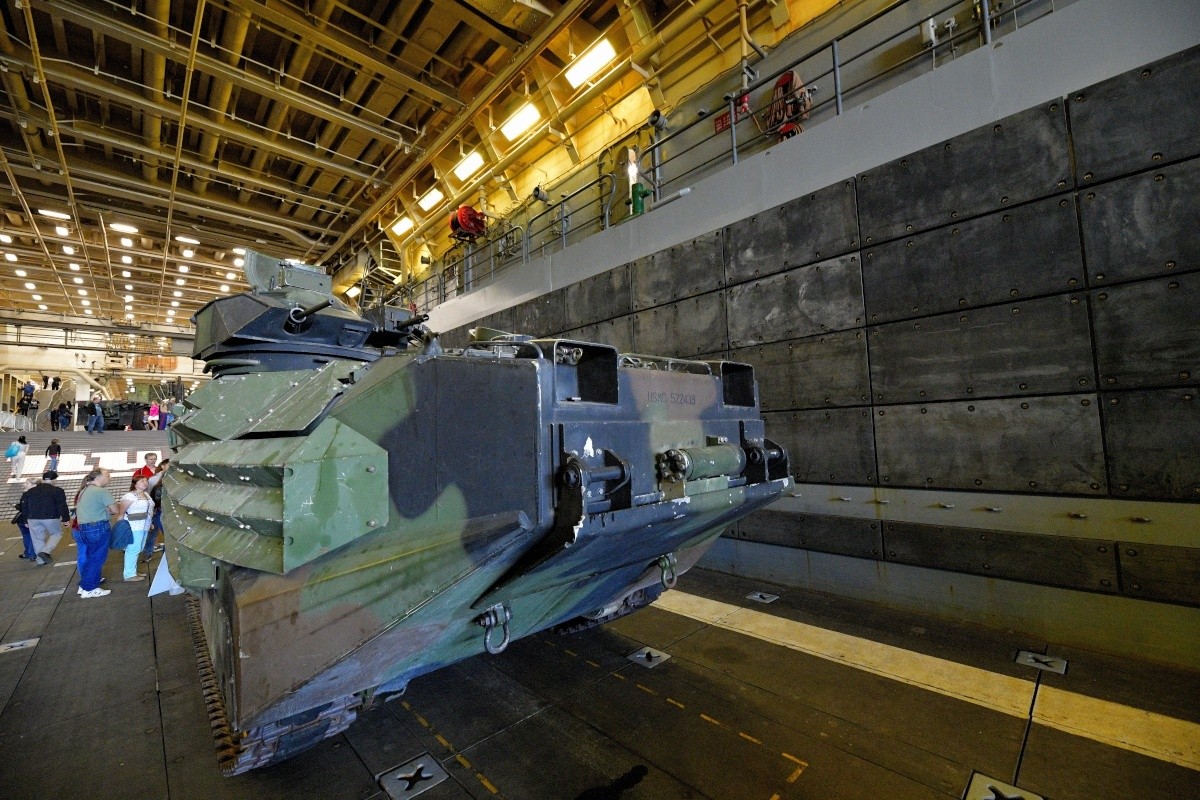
xmin=140 ymin=458 xmax=169 ymax=562
xmin=143 ymin=397 xmax=176 ymax=431
xmin=22 ymin=471 xmax=70 ymax=567
xmin=71 ymin=475 xmax=106 ymax=582
xmin=51 ymin=401 xmax=74 ymax=431
xmin=19 ymin=381 xmax=35 ymax=416
xmin=88 ymin=395 xmax=105 ymax=434
xmin=12 ymin=478 xmax=41 ymax=561
xmin=130 ymin=453 xmax=164 ymax=552
xmin=118 ymin=476 xmax=155 ymax=582
xmin=76 ymin=468 xmax=120 ymax=599
xmin=46 ymin=439 xmax=61 ymax=474
xmin=42 ymin=376 xmax=62 ymax=390
xmin=10 ymin=436 xmax=29 ymax=480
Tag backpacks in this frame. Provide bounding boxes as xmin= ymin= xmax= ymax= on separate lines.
xmin=150 ymin=481 xmax=162 ymax=509
xmin=58 ymin=416 xmax=64 ymax=421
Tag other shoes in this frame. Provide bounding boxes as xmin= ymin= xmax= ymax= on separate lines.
xmin=98 ymin=432 xmax=104 ymax=433
xmin=67 ymin=427 xmax=69 ymax=430
xmin=38 ymin=552 xmax=53 ymax=563
xmin=153 ymin=544 xmax=163 ymax=552
xmin=138 ymin=572 xmax=148 ymax=576
xmin=139 ymin=555 xmax=152 ymax=563
xmin=52 ymin=430 xmax=56 ymax=431
xmin=61 ymin=429 xmax=64 ymax=432
xmin=77 ymin=586 xmax=83 ymax=595
xmin=12 ymin=475 xmax=17 ymax=478
xmin=30 ymin=558 xmax=36 ymax=561
xmin=100 ymin=578 xmax=106 ymax=582
xmin=89 ymin=432 xmax=94 ymax=435
xmin=37 ymin=562 xmax=47 ymax=567
xmin=81 ymin=588 xmax=112 ymax=599
xmin=124 ymin=576 xmax=145 ymax=581
xmin=19 ymin=554 xmax=29 ymax=559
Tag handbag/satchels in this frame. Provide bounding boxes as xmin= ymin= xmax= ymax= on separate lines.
xmin=11 ymin=512 xmax=22 ymax=524
xmin=5 ymin=444 xmax=20 ymax=457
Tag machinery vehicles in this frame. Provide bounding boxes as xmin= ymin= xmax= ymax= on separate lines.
xmin=159 ymin=252 xmax=794 ymax=778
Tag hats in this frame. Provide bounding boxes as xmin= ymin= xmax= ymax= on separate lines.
xmin=43 ymin=471 xmax=59 ymax=480
